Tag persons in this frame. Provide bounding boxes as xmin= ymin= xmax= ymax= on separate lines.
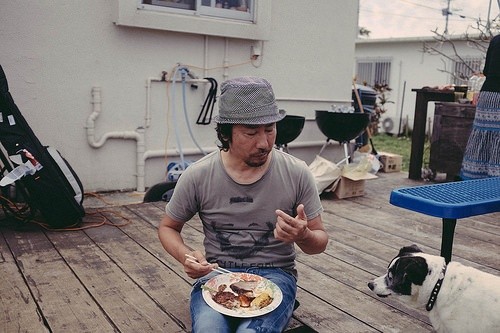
xmin=158 ymin=75 xmax=328 ymax=332
xmin=459 ymin=32 xmax=500 ymax=181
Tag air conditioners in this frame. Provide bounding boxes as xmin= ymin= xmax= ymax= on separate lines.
xmin=381 ymin=114 xmax=405 ymax=134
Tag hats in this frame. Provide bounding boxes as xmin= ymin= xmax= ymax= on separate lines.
xmin=214 ymin=76 xmax=287 ymax=125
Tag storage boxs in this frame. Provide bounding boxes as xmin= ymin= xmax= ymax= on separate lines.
xmin=324 ymin=171 xmax=380 ymax=200
xmin=381 ymin=153 xmax=403 ymax=173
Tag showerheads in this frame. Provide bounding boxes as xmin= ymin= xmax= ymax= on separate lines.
xmin=187 ymin=71 xmax=199 ymax=88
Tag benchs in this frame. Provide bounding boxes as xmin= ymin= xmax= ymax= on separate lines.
xmin=389 ymin=176 xmax=500 ymax=262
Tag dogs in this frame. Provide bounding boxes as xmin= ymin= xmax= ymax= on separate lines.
xmin=367 ymin=244 xmax=500 ymax=333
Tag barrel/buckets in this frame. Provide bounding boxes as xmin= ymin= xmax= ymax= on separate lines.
xmin=349 ymin=80 xmax=376 ymax=148
xmin=429 ymin=101 xmax=476 ymax=179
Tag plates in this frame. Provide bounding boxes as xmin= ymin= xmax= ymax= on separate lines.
xmin=202 ymin=272 xmax=283 ymax=317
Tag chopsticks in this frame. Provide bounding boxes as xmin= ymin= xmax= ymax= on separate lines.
xmin=184 ymin=254 xmax=248 ymax=284
